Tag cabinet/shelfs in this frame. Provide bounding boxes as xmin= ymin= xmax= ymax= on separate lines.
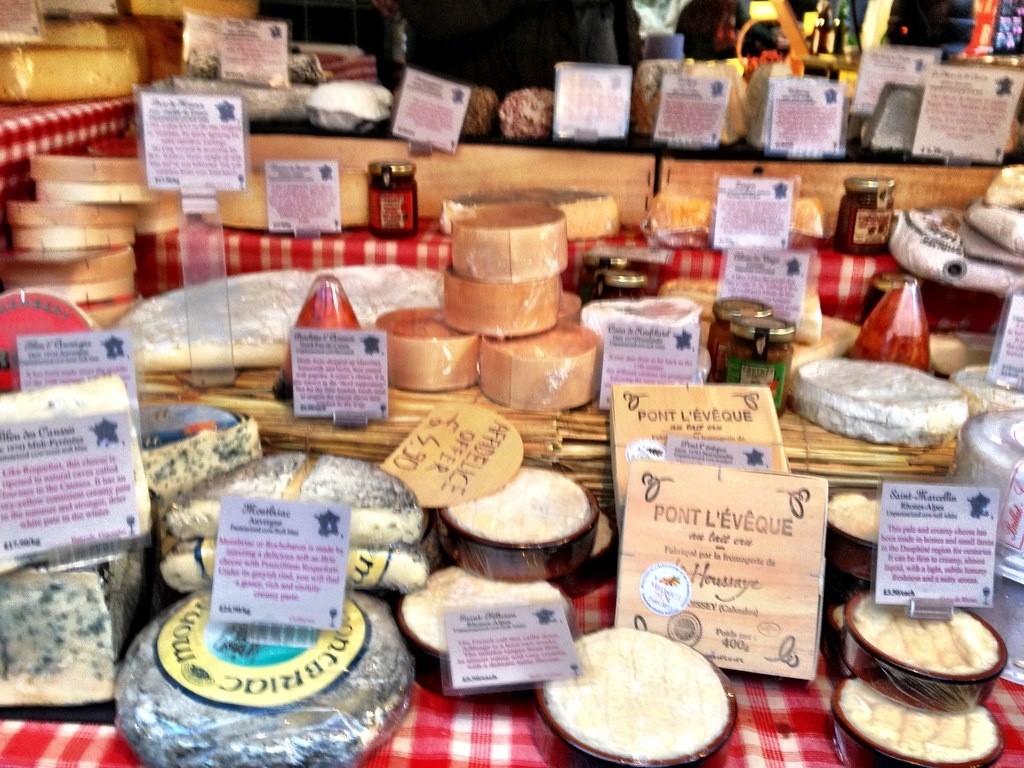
xmin=0 ymin=97 xmax=1024 ymax=767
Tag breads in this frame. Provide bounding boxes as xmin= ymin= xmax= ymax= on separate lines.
xmin=0 ymin=0 xmax=251 ymax=105
xmin=364 ymin=187 xmax=619 ymax=413
xmin=0 ymin=375 xmax=431 ymax=768
xmin=644 ymin=193 xmax=1001 ymax=379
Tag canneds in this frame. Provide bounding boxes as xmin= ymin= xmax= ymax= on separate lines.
xmin=834 ymin=176 xmax=896 ymax=255
xmin=860 ymin=273 xmax=907 ymax=323
xmin=576 ymin=256 xmax=648 ymax=304
xmin=705 ymin=299 xmax=795 ymax=419
xmin=366 ymin=160 xmax=419 ymax=238
